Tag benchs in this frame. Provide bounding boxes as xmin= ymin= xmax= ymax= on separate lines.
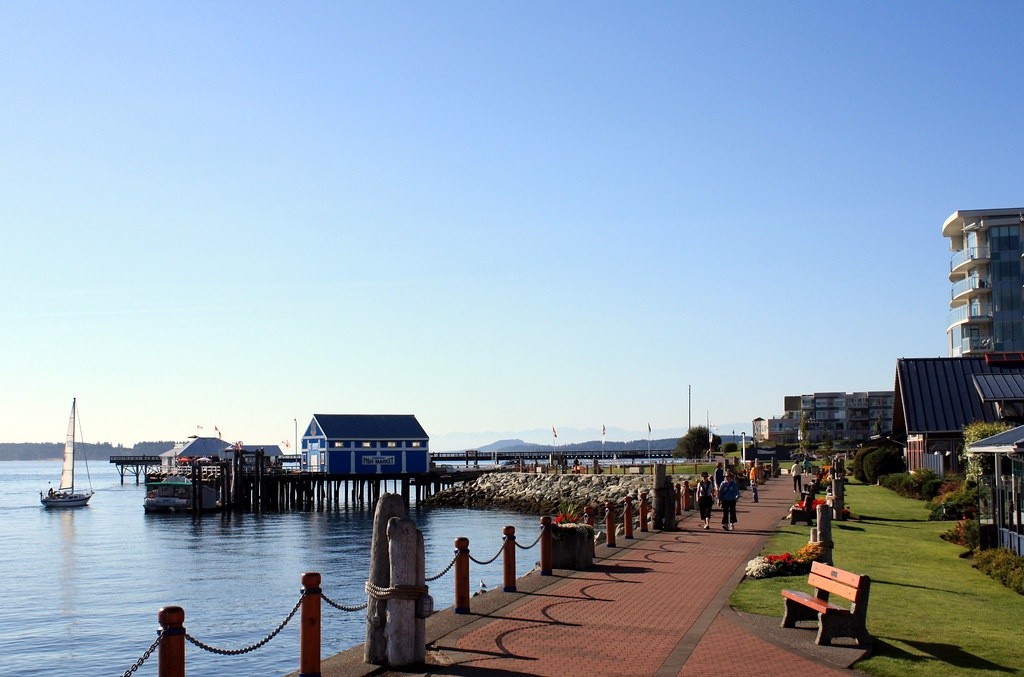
xmin=791 ymin=473 xmax=821 ymax=526
xmin=780 ymin=560 xmax=873 ymax=648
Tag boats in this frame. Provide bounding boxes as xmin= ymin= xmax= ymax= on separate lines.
xmin=141 ymin=478 xmax=193 ymax=517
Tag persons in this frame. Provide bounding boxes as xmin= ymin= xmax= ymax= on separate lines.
xmin=718 ymin=472 xmax=740 ymax=530
xmin=48 ymin=488 xmax=55 ymax=497
xmin=803 ymin=457 xmax=810 ymax=478
xmin=714 ymin=462 xmax=723 ymax=505
xmin=183 ymin=462 xmax=187 ymax=466
xmin=574 ymin=457 xmax=578 ymax=465
xmin=748 ymin=462 xmax=758 ymax=503
xmin=695 ymin=472 xmax=715 ymax=530
xmin=791 ymin=460 xmax=802 ymax=493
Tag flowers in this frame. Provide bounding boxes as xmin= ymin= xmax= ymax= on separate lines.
xmin=552 ymin=500 xmax=585 ymax=524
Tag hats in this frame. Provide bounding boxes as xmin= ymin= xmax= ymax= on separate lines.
xmin=723 ymin=472 xmax=734 ymax=476
xmin=701 ymin=472 xmax=709 ymax=477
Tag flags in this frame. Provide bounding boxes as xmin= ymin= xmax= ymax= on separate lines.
xmin=648 ymin=423 xmax=651 ymax=432
xmin=603 ymin=425 xmax=606 ymax=435
xmin=711 ymin=423 xmax=717 ymax=430
xmin=197 ymin=425 xmax=203 ymax=429
xmin=553 ymin=427 xmax=557 ymax=437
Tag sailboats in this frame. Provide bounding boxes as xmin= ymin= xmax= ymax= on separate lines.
xmin=40 ymin=397 xmax=96 ymax=507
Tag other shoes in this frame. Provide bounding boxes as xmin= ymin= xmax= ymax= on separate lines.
xmin=722 ymin=526 xmax=729 ymax=531
xmin=704 ymin=525 xmax=709 ymax=529
xmin=730 ymin=525 xmax=734 ymax=530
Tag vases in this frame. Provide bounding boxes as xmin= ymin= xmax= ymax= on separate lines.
xmin=549 ymin=523 xmax=595 ymax=570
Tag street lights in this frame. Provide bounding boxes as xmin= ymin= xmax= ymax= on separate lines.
xmin=741 ymin=432 xmax=746 ymax=465
xmin=294 ymin=419 xmax=299 ymax=470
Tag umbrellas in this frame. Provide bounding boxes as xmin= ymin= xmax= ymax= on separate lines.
xmin=197 ymin=457 xmax=212 ymax=461
xmin=180 ymin=457 xmax=190 ymax=462
